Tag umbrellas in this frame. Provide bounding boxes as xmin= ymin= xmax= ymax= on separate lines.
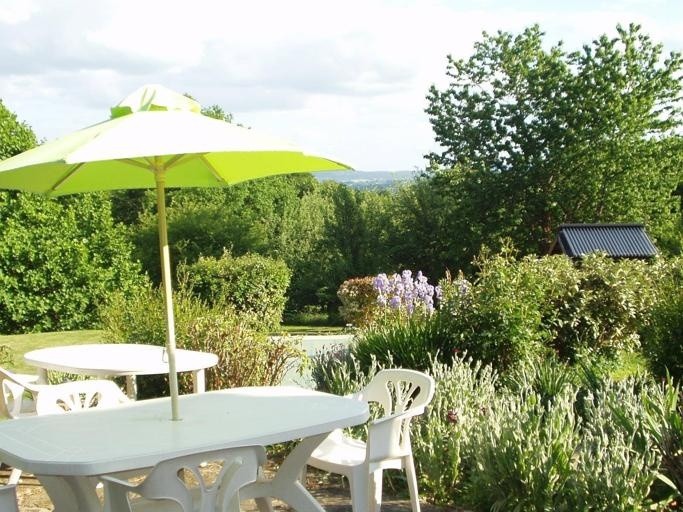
xmin=0 ymin=83 xmax=356 ymax=422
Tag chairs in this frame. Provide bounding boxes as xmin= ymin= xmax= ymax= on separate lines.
xmin=36 ymin=379 xmax=154 ymax=489
xmin=298 ymin=369 xmax=435 ymax=512
xmin=97 ymin=445 xmax=269 ymax=512
xmin=0 ymin=484 xmax=19 ymax=512
xmin=0 ymin=368 xmax=71 ymax=486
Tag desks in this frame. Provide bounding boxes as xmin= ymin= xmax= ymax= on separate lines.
xmin=0 ymin=385 xmax=369 ymax=512
xmin=24 ymin=344 xmax=218 ymax=401
